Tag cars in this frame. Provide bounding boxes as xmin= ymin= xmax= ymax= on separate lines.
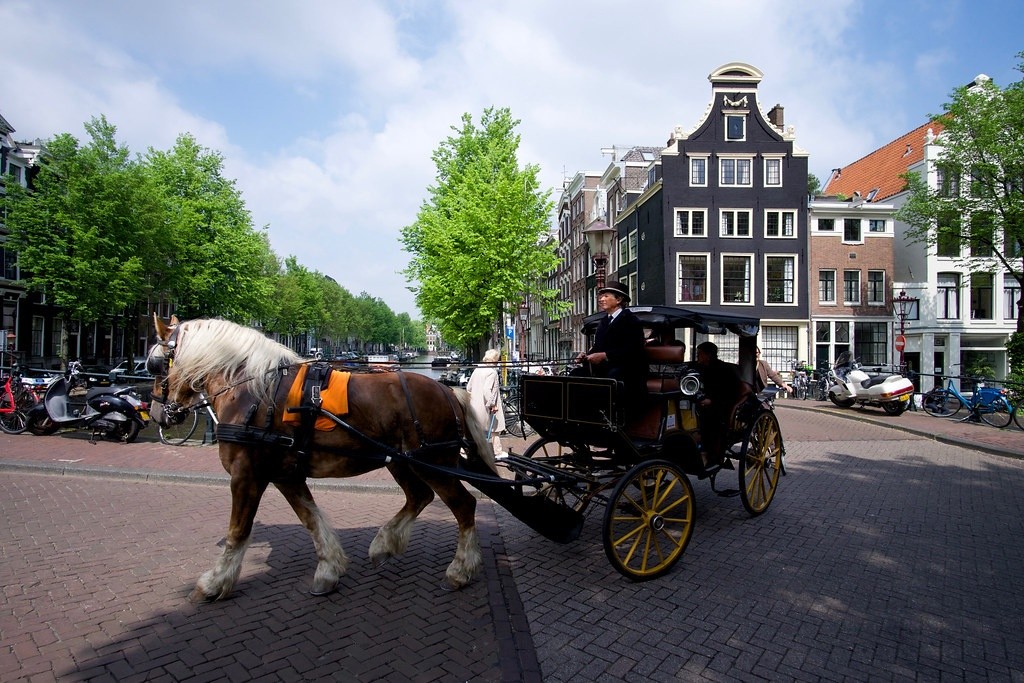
xmin=66 ymin=366 xmax=110 ymax=388
xmin=109 ymin=360 xmax=152 ymax=382
xmin=22 ymin=368 xmax=53 ymax=391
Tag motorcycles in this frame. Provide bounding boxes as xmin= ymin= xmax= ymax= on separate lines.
xmin=827 ymin=352 xmax=916 ymax=416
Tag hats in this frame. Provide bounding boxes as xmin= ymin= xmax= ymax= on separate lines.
xmin=598 ymin=281 xmax=631 ymax=303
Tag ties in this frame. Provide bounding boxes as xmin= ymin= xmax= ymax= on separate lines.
xmin=608 ymin=316 xmax=613 ymax=323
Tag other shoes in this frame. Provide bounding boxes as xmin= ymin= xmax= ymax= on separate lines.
xmin=495 ymin=452 xmax=508 ymax=461
xmin=702 ymin=456 xmax=709 ymax=468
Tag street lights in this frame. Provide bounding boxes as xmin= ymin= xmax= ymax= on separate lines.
xmin=891 ymin=288 xmax=916 ymax=373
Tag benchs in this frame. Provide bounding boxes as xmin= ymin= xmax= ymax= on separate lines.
xmin=642 ymin=346 xmax=684 ymax=394
xmin=682 ymin=361 xmax=740 ymax=430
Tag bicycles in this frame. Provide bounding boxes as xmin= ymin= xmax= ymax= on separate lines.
xmin=0 ymin=363 xmax=41 ymax=432
xmin=790 ymin=360 xmax=829 ymax=402
xmin=753 ymin=388 xmax=787 ymax=476
xmin=158 ymin=373 xmax=221 ymax=446
xmin=1011 ymin=397 xmax=1024 ymax=430
xmin=500 ymin=383 xmax=535 ymax=436
xmin=921 ymin=363 xmax=1013 ymax=429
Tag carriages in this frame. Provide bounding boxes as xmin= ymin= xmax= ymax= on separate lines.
xmin=147 ymin=305 xmax=786 ymax=602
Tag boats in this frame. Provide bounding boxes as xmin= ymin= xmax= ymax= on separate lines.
xmin=336 ymin=350 xmax=471 ymax=387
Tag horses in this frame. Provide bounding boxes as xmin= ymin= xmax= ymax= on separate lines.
xmin=146 ymin=313 xmax=498 ymax=603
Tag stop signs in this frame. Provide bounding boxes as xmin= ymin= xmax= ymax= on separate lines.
xmin=896 ymin=335 xmax=907 ymax=352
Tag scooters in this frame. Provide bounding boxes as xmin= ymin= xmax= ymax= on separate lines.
xmin=25 ymin=361 xmax=150 ymax=444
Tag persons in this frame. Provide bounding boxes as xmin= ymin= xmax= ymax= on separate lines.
xmin=467 ymin=350 xmax=508 ymax=458
xmin=755 ymin=346 xmax=793 ymax=450
xmin=566 ymin=279 xmax=650 ymax=427
xmin=649 ymin=341 xmax=732 ymax=449
